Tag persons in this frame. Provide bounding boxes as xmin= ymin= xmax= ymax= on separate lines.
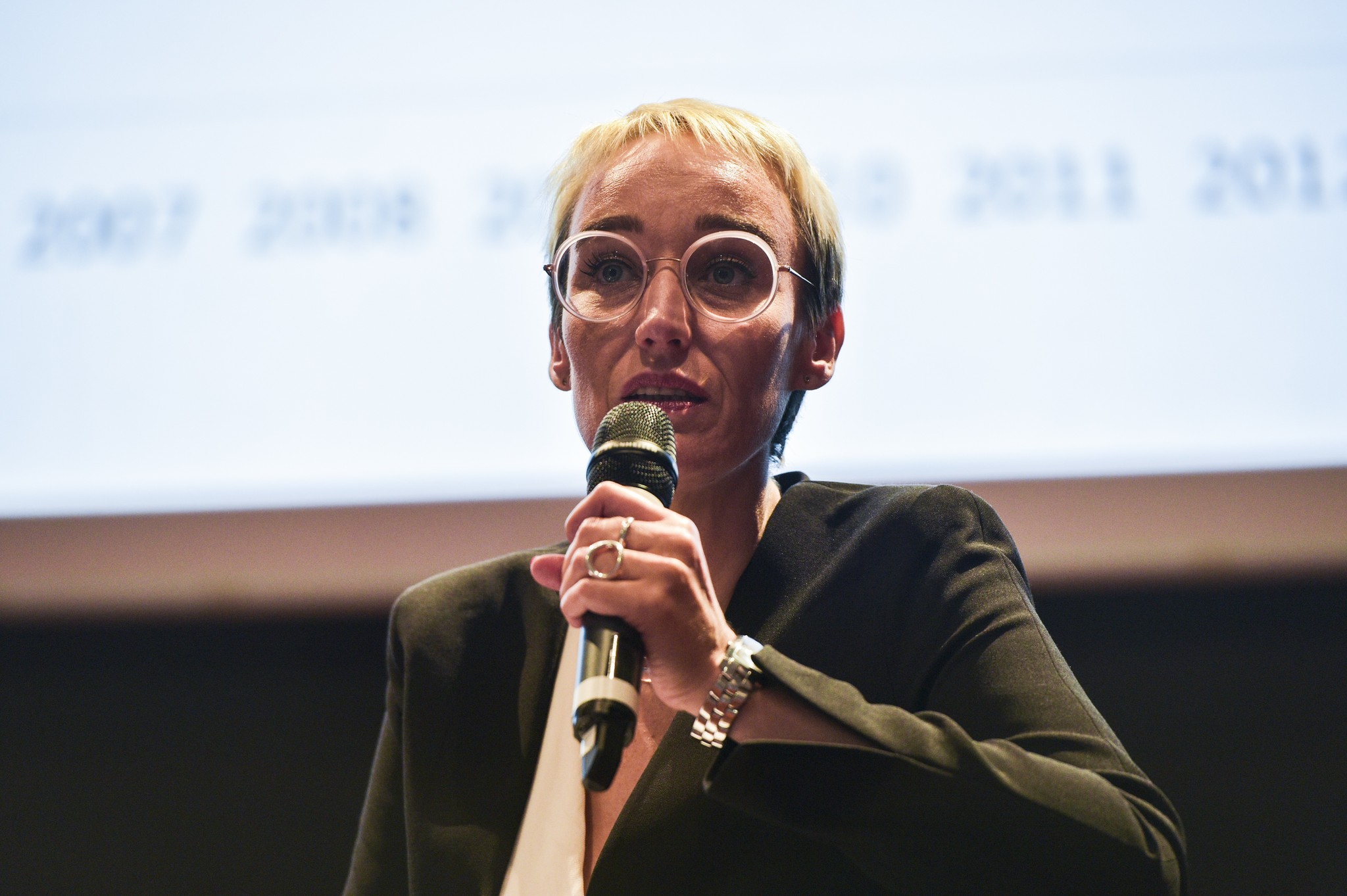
xmin=357 ymin=96 xmax=1186 ymax=896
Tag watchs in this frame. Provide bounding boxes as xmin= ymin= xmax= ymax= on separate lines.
xmin=691 ymin=635 xmax=765 ymax=748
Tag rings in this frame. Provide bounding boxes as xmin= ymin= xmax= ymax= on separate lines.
xmin=585 ymin=539 xmax=624 ymax=580
xmin=618 ymin=516 xmax=635 ymax=548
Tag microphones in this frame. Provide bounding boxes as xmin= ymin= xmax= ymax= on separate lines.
xmin=569 ymin=400 xmax=682 ymax=795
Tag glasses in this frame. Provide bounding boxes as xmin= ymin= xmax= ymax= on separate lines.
xmin=543 ymin=230 xmax=815 ymax=323
